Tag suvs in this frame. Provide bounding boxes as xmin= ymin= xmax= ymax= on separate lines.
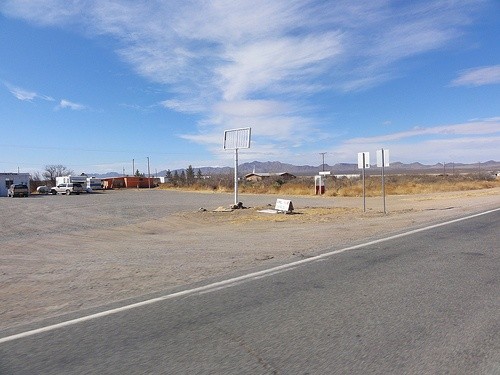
xmin=7 ymin=184 xmax=29 ymax=198
xmin=50 ymin=183 xmax=83 ymax=195
